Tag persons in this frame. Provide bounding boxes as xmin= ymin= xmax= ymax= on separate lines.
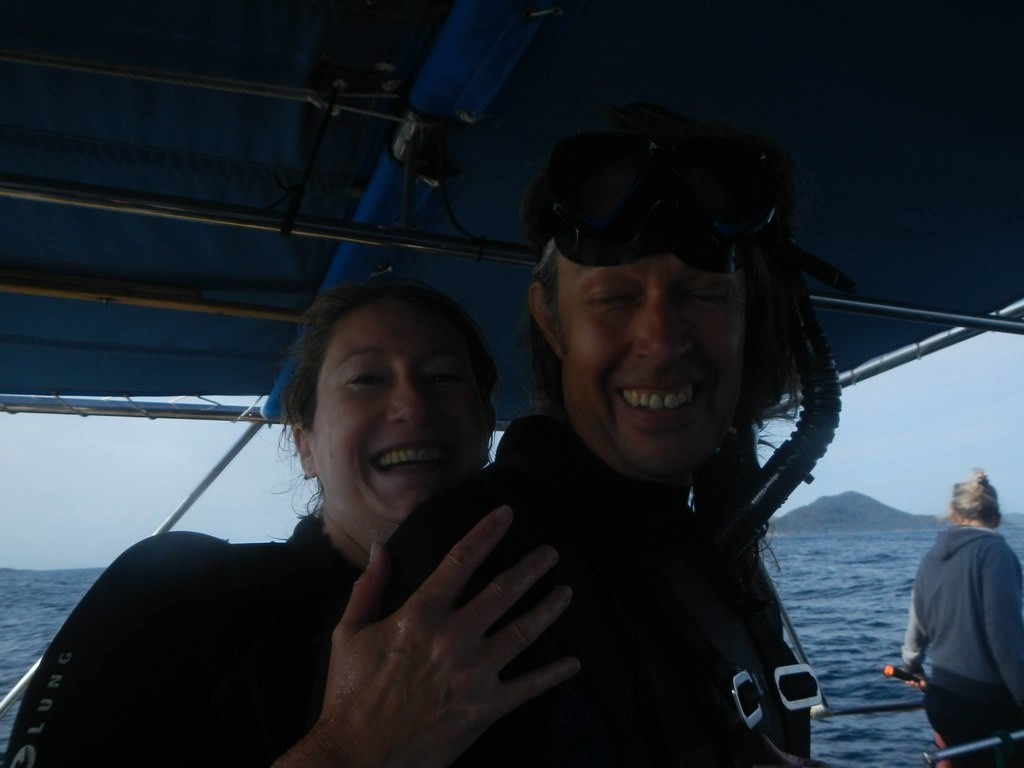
xmin=361 ymin=98 xmax=813 ymax=768
xmin=901 ymin=470 xmax=1024 ymax=768
xmin=0 ymin=277 xmax=583 ymax=767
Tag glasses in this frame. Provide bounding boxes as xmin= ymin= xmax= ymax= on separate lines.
xmin=532 ymin=137 xmax=767 ymax=270
